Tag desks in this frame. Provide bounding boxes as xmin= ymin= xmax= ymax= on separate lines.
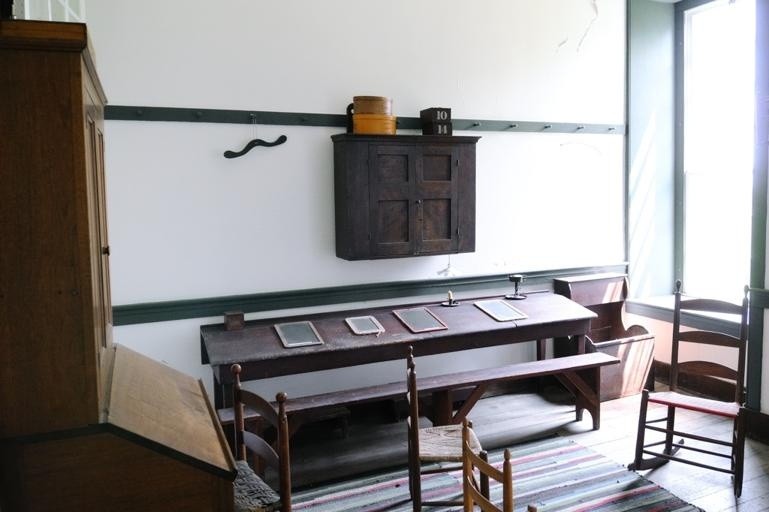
xmin=201 ymin=293 xmax=598 ymax=464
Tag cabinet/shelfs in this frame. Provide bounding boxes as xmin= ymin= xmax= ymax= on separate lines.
xmin=0 ymin=19 xmax=113 ymax=436
xmin=333 ymin=136 xmax=479 ymax=261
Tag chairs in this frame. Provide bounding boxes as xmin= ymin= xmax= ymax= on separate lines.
xmin=403 ymin=344 xmax=492 ymax=510
xmin=460 ymin=416 xmax=539 ymax=512
xmin=628 ymin=279 xmax=749 ymax=497
xmin=228 ymin=365 xmax=295 ymax=512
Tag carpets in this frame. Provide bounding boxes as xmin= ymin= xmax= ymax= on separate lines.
xmin=291 ymin=432 xmax=704 ymax=512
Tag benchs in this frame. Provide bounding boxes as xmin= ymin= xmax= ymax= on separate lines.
xmin=220 ymin=350 xmax=625 ymax=483
